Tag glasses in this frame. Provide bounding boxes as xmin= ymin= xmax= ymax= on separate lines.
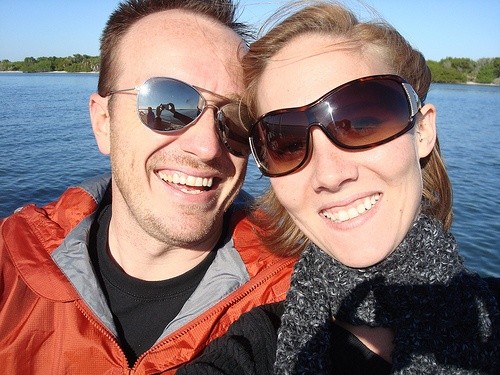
xmin=248 ymin=74 xmax=425 ymax=177
xmin=105 ymin=76 xmax=254 ymax=158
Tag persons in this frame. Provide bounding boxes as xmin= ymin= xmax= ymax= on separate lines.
xmin=147 ymin=107 xmax=158 ymax=128
xmin=155 ymin=103 xmax=195 ymax=130
xmin=0 ymin=1 xmax=314 ymax=375
xmin=174 ymin=0 xmax=499 ymax=375
